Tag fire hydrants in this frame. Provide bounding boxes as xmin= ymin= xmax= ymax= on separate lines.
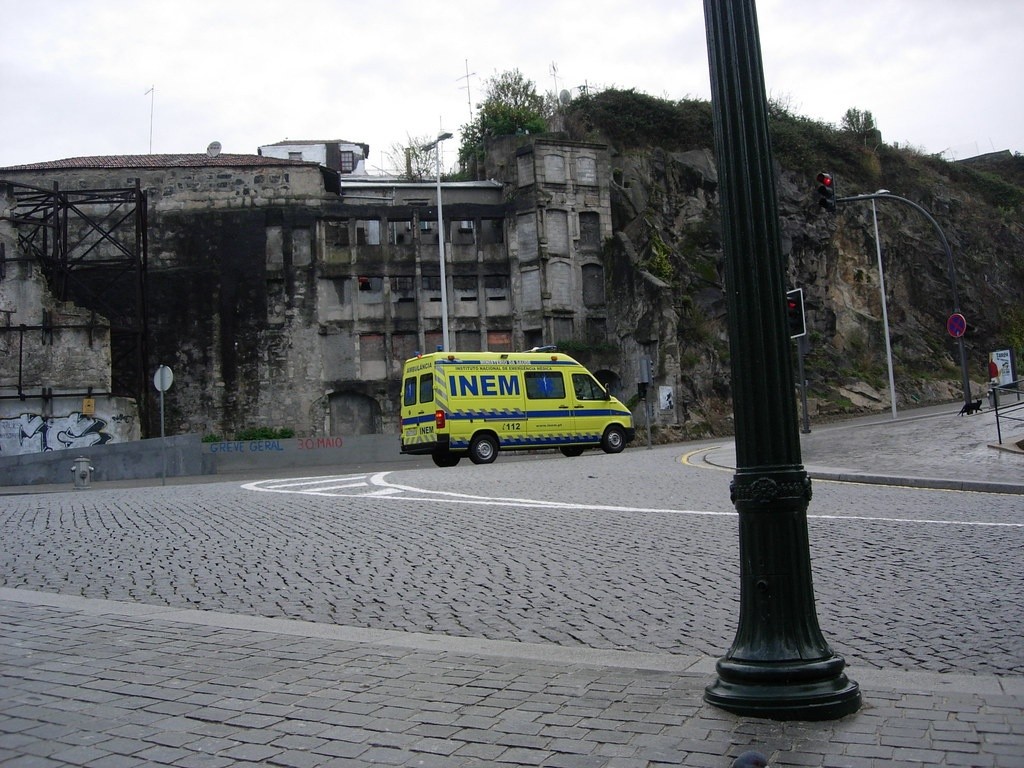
xmin=71 ymin=455 xmax=94 ymax=491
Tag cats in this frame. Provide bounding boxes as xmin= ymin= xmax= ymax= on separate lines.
xmin=957 ymin=400 xmax=983 ymax=417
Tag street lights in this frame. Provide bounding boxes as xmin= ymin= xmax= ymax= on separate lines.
xmin=420 ymin=131 xmax=453 ymax=352
xmin=871 ymin=189 xmax=897 ymax=419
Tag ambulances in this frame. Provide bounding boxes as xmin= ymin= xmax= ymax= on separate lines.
xmin=398 ymin=344 xmax=636 ymax=467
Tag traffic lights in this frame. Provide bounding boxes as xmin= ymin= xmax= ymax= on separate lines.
xmin=816 ymin=170 xmax=837 ymax=212
xmin=785 ymin=288 xmax=807 ymax=339
